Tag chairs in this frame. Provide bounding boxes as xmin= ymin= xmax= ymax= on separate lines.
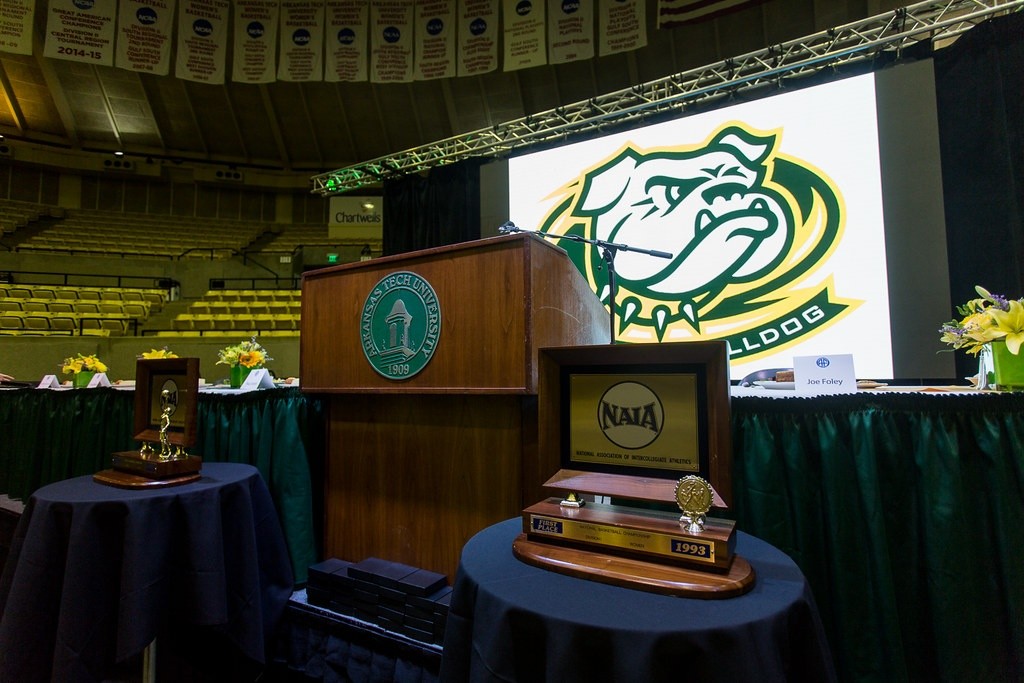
xmin=0 ymin=199 xmax=382 ymax=338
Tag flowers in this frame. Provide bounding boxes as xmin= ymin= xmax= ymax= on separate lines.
xmin=933 ymin=285 xmax=1024 ymax=358
xmin=57 ymin=352 xmax=111 ymax=375
xmin=215 ymin=336 xmax=273 ymax=369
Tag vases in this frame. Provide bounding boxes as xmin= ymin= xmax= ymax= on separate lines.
xmin=992 ymin=342 xmax=1024 ymax=391
xmin=73 ymin=371 xmax=96 ymax=388
xmin=230 ymin=363 xmax=261 ymax=388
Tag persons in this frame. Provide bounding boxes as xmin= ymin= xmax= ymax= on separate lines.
xmin=158 ymin=388 xmax=174 ymax=462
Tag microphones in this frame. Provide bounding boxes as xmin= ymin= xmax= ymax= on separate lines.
xmin=499 ymin=221 xmax=515 ymax=231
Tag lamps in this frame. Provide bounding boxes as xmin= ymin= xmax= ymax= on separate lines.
xmin=359 ymin=244 xmax=374 ymax=262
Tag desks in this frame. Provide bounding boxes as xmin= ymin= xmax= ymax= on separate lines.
xmin=436 ymin=516 xmax=837 ymax=683
xmin=0 ymin=381 xmax=312 ymax=586
xmin=0 ymin=462 xmax=296 ymax=683
xmin=731 ymin=386 xmax=1024 ymax=683
